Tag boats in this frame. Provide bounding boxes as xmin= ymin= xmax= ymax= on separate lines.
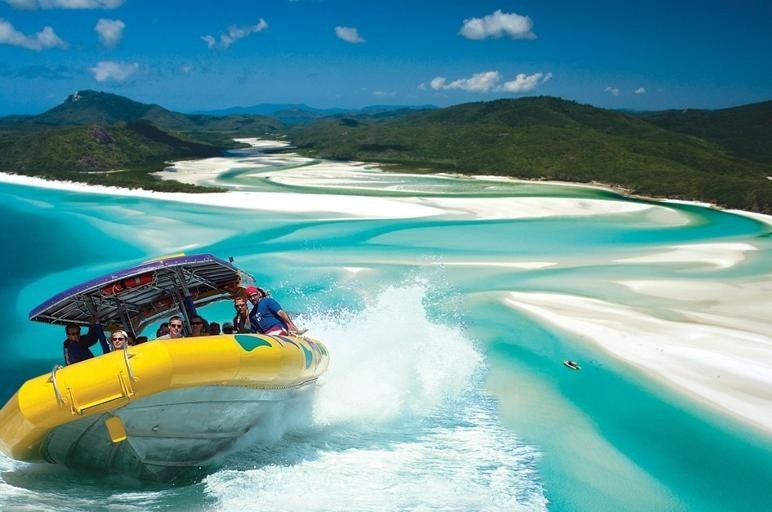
xmin=563 ymin=360 xmax=580 ymax=370
xmin=0 ymin=253 xmax=329 ymax=486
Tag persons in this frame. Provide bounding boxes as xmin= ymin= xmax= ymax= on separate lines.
xmin=186 ymin=315 xmax=209 ymax=337
xmin=126 ymin=332 xmax=136 ymax=346
xmin=135 ymin=336 xmax=147 ymax=345
xmin=256 ymin=287 xmax=299 ymax=334
xmin=155 ymin=316 xmax=184 ymax=340
xmin=62 ymin=314 xmax=99 ymax=367
xmin=109 ymin=329 xmax=130 ymax=350
xmin=221 ymin=322 xmax=233 ymax=334
xmin=232 ymin=296 xmax=250 ymax=333
xmin=243 ymin=286 xmax=296 ymax=336
xmin=207 ymin=322 xmax=220 ymax=335
xmin=157 ymin=322 xmax=169 ymax=338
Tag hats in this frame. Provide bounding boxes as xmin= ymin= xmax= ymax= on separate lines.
xmin=223 ymin=323 xmax=233 ymax=329
xmin=244 ymin=287 xmax=257 ymax=295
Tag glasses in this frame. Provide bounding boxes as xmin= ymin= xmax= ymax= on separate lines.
xmin=236 ymin=305 xmax=244 ymax=307
xmin=248 ymin=294 xmax=257 ymax=298
xmin=172 ymin=324 xmax=181 ymax=328
xmin=67 ymin=332 xmax=80 ymax=335
xmin=190 ymin=321 xmax=202 ymax=325
xmin=113 ymin=338 xmax=123 ymax=341
